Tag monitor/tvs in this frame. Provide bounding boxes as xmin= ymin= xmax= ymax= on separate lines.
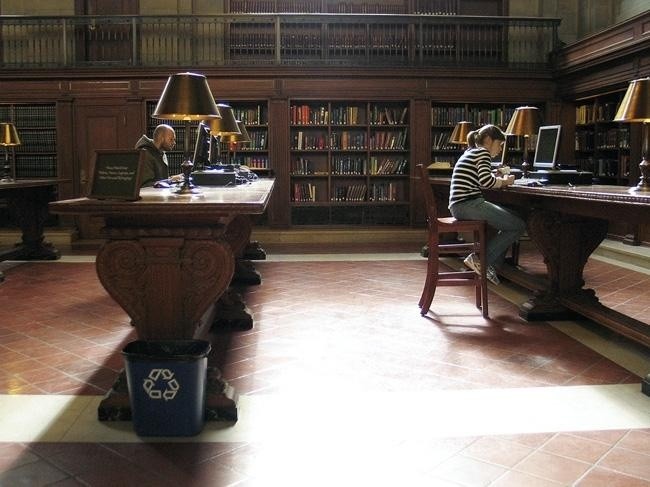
xmin=533 ymin=122 xmax=562 ymax=168
xmin=491 ymin=135 xmax=508 ymax=167
xmin=209 ymin=134 xmax=220 ymax=164
xmin=193 ymin=122 xmax=210 ymax=170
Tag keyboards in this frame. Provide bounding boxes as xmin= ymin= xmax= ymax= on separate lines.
xmin=514 ymin=179 xmax=548 ymax=187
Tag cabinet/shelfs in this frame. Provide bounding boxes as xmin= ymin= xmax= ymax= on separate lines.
xmin=272 ymin=96 xmax=431 ymax=228
xmin=570 ymin=88 xmax=643 ymax=247
xmin=207 ymin=98 xmax=273 ymax=174
xmin=145 ymin=98 xmax=198 ymax=180
xmin=410 ymin=101 xmax=553 ymax=229
xmin=0 ymin=100 xmax=74 ymax=229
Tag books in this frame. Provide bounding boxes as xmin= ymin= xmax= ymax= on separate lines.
xmin=576 ymin=100 xmax=650 ymax=179
xmin=431 ymin=104 xmax=536 ymax=170
xmin=147 ymin=101 xmax=409 ymax=203
xmin=0 ymin=105 xmax=56 ymax=179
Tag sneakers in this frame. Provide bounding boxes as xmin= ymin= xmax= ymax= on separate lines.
xmin=464 ymin=252 xmax=500 ymax=287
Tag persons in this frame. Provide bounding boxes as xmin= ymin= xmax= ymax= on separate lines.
xmin=133 ymin=123 xmax=176 ymax=199
xmin=448 ymin=124 xmax=528 ymax=286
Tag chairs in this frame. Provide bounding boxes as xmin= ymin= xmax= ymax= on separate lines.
xmin=415 ymin=162 xmax=488 ymax=318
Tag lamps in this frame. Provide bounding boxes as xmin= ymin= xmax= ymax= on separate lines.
xmin=504 ymin=106 xmax=541 ymax=178
xmin=0 ymin=122 xmax=21 ymax=183
xmin=150 ymin=72 xmax=223 ymax=195
xmin=219 ymin=120 xmax=252 ymax=165
xmin=447 ymin=122 xmax=476 ymax=153
xmin=611 ymin=78 xmax=650 ymax=192
xmin=196 ymin=103 xmax=242 ymax=166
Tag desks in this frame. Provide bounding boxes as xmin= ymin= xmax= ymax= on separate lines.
xmin=47 ymin=177 xmax=276 ymax=421
xmin=0 ymin=177 xmax=72 ymax=284
xmin=407 ymin=174 xmax=650 ymax=397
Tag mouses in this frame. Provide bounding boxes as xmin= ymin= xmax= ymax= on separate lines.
xmin=154 ymin=182 xmax=170 ymax=187
xmin=528 ymin=182 xmax=543 ymax=187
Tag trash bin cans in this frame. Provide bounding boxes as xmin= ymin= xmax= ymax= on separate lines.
xmin=121 ymin=339 xmax=212 ymax=437
